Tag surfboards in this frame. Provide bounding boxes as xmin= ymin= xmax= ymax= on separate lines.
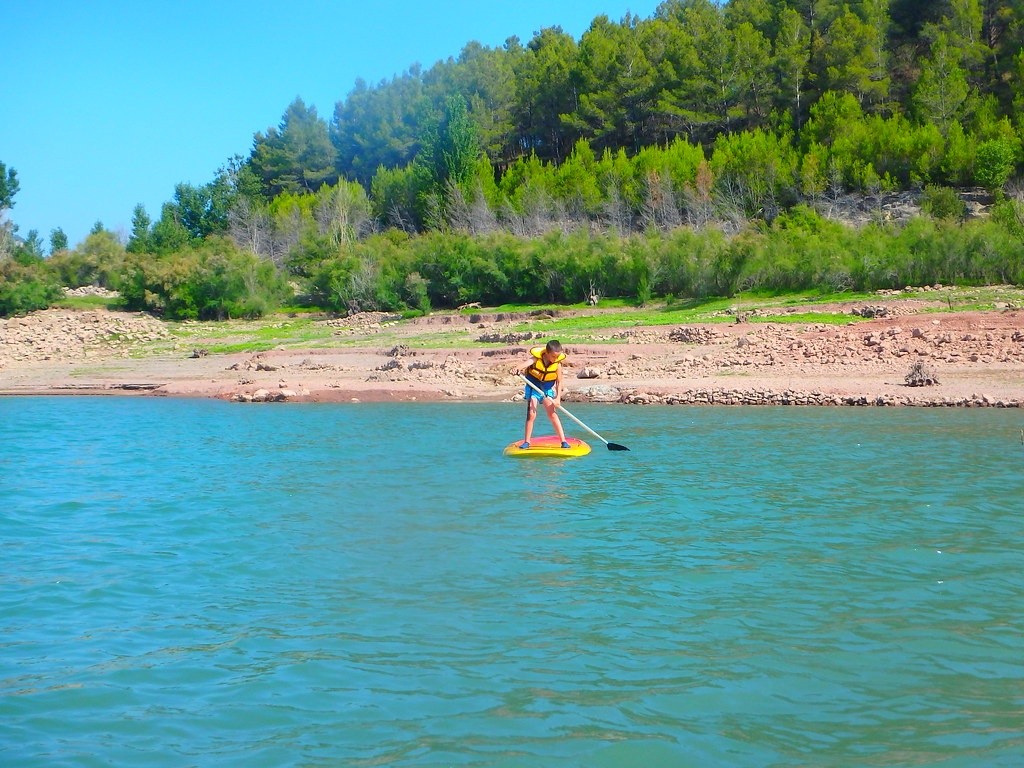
xmin=503 ymin=434 xmax=592 ymax=458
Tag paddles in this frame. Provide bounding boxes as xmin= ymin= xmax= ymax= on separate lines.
xmin=514 ymin=369 xmax=631 ymax=451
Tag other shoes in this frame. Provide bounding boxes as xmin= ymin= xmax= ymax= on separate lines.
xmin=519 ymin=442 xmax=529 ymax=448
xmin=561 ymin=442 xmax=570 ymax=448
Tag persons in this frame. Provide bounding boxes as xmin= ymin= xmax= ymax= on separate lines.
xmin=510 ymin=340 xmax=571 ymax=449
